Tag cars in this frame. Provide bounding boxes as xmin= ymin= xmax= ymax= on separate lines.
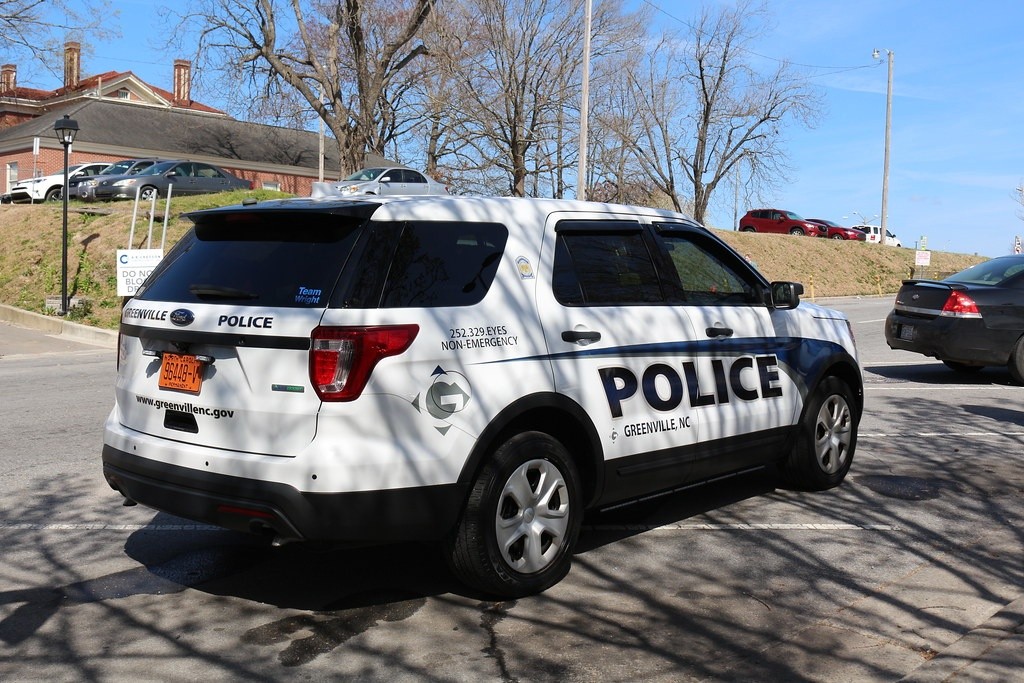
xmin=804 ymin=219 xmax=866 ymax=241
xmin=99 ymin=194 xmax=863 ymax=599
xmin=739 ymin=209 xmax=828 ymax=237
xmin=95 ymin=160 xmax=253 ymax=200
xmin=332 ymin=166 xmax=448 ymax=195
xmin=68 ymin=160 xmax=165 ymax=202
xmin=884 ymin=254 xmax=1024 ymax=388
xmin=11 ymin=162 xmax=114 ymax=203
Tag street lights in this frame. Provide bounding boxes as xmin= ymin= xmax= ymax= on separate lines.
xmin=872 ymin=47 xmax=893 ymax=246
xmin=53 ymin=114 xmax=82 ymax=312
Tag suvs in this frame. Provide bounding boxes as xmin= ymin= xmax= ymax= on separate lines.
xmin=852 ymin=225 xmax=901 ymax=247
